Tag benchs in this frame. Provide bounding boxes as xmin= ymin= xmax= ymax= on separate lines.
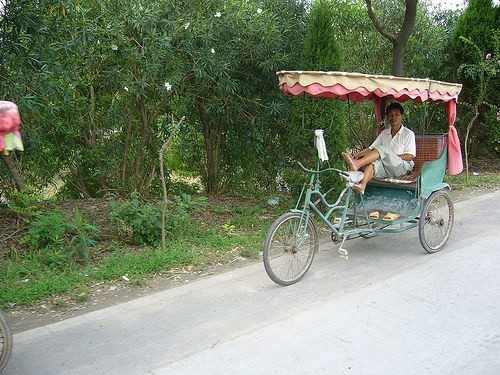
xmin=360 ymin=133 xmax=448 ymax=190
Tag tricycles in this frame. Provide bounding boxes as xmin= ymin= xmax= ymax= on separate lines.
xmin=263 ymin=72 xmax=464 ymax=286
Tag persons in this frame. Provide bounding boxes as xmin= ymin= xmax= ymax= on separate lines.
xmin=341 ymin=102 xmax=416 ymax=194
